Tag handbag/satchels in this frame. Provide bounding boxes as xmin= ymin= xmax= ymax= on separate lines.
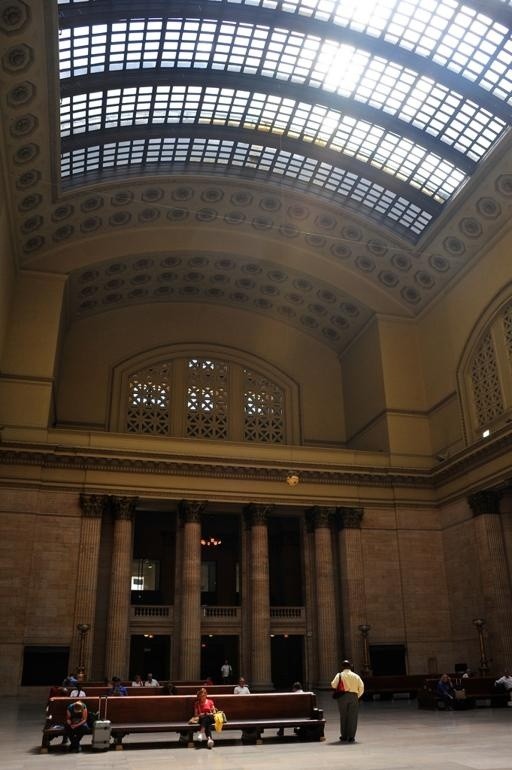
xmin=332 ymin=673 xmax=344 ymax=700
xmin=210 ymin=708 xmax=227 ymax=723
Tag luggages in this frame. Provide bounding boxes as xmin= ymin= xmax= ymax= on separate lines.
xmin=92 ymin=695 xmax=112 ymax=753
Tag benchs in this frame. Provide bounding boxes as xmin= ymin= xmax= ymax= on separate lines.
xmin=419 ymin=678 xmax=511 ymax=711
xmin=355 ymin=672 xmax=463 ymax=702
xmin=42 ymin=681 xmax=325 ymax=747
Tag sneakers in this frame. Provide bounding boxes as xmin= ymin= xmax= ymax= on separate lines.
xmin=197 ymin=735 xmax=202 ymax=742
xmin=208 ymin=739 xmax=214 ymax=748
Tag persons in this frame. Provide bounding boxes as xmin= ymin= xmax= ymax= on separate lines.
xmin=193 ymin=688 xmax=217 ymax=748
xmin=276 ymin=682 xmax=305 ymax=736
xmin=206 ymin=676 xmax=214 ymax=685
xmin=220 ymin=660 xmax=233 ymax=685
xmin=435 ymin=673 xmax=455 ymax=711
xmin=61 ymin=672 xmax=179 ymax=753
xmin=233 ymin=676 xmax=251 ymax=694
xmin=462 ymin=668 xmax=471 ymax=678
xmin=330 ymin=658 xmax=366 ymax=742
xmin=494 ymin=669 xmax=512 ymax=707
